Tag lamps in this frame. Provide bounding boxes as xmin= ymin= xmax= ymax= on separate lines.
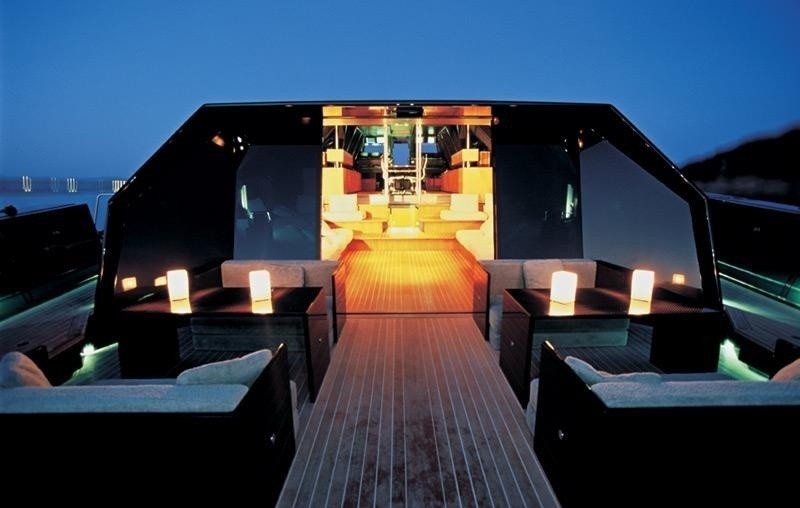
xmin=550 ymin=270 xmax=577 ymax=304
xmin=631 ymin=268 xmax=655 ymax=301
xmin=249 ymin=270 xmax=272 ymax=302
xmin=166 ymin=268 xmax=190 ymax=301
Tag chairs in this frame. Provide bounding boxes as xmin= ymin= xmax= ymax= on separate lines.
xmin=441 ymin=193 xmax=488 ymax=221
xmin=325 ymin=193 xmax=366 ymax=222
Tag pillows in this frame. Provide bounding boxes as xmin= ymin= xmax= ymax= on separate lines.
xmin=0 ymin=350 xmax=52 ymax=387
xmin=523 ymin=259 xmax=563 ymax=289
xmin=768 ymin=359 xmax=800 ymax=382
xmin=564 ymin=355 xmax=661 ymax=384
xmin=173 ymin=348 xmax=272 ymax=386
xmin=321 ymin=219 xmax=330 ymax=236
xmin=221 ymin=259 xmax=305 ymax=287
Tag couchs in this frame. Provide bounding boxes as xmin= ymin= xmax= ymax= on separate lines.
xmin=0 ymin=341 xmax=300 ymax=508
xmin=321 ymin=218 xmax=353 ymax=261
xmin=524 ymin=338 xmax=800 ymax=508
xmin=456 ymin=216 xmax=494 ymax=260
xmin=472 ymin=258 xmax=633 ymax=350
xmin=181 ymin=261 xmax=347 ymax=348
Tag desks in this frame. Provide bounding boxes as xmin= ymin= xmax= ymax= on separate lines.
xmin=116 ymin=285 xmax=331 ymax=403
xmin=499 ymin=287 xmax=723 ymax=408
